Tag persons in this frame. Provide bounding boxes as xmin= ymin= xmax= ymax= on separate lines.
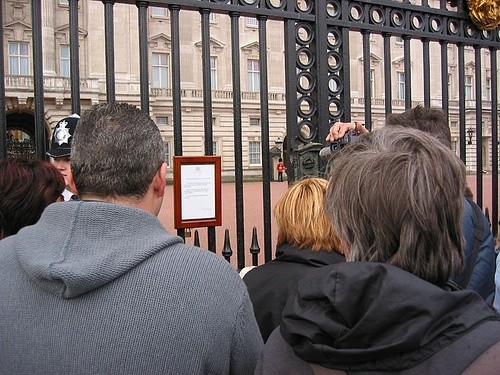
xmin=238 ymin=177 xmax=350 ymax=344
xmin=45 ymin=116 xmax=80 ymax=201
xmin=325 ymin=105 xmax=497 ymax=309
xmin=1 ymin=103 xmax=264 ymax=375
xmin=276 ymin=158 xmax=287 ymax=182
xmin=253 ymin=124 xmax=499 ymax=375
xmin=0 ymin=157 xmax=66 ymax=238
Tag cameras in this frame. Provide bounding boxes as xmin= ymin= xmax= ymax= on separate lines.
xmin=319 ymin=132 xmax=361 ymax=161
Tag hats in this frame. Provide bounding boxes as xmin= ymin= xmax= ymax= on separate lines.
xmin=45 ymin=113 xmax=81 ymax=158
